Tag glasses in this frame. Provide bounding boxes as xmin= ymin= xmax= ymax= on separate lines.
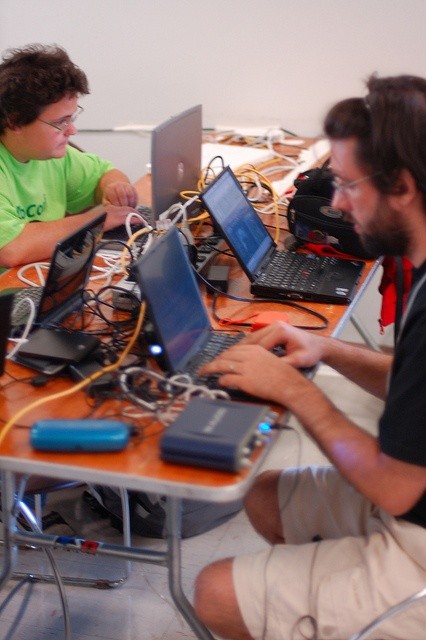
xmin=36 ymin=104 xmax=84 ymax=130
xmin=331 ymin=175 xmax=371 ymax=193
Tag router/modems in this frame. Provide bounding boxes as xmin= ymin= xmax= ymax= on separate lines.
xmin=161 ymin=396 xmax=272 ymax=474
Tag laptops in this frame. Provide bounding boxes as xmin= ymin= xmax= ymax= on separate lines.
xmin=104 ymin=105 xmax=201 ymax=238
xmin=134 ymin=227 xmax=287 ymax=405
xmin=200 ymin=166 xmax=365 ymax=303
xmin=0 ymin=212 xmax=107 ymax=329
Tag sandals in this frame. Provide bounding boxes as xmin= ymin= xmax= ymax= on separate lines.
xmin=15 ymin=507 xmax=79 ymax=537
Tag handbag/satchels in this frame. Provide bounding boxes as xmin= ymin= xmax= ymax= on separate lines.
xmin=286 ymin=166 xmax=380 ymax=260
xmin=81 ymin=481 xmax=243 ymax=539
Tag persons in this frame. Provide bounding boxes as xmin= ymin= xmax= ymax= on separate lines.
xmin=192 ymin=72 xmax=426 ymax=640
xmin=1 ymin=43 xmax=150 ymax=541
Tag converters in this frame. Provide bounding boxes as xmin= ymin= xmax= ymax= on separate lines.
xmin=69 ymin=361 xmax=113 ymax=396
xmin=207 ymin=266 xmax=229 ymax=294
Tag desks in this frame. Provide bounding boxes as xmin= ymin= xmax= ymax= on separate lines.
xmin=0 ymin=131 xmax=383 ymax=640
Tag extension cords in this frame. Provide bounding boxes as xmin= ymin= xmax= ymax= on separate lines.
xmin=185 ymin=234 xmax=224 ymax=283
xmin=112 ymin=234 xmax=153 ymax=311
